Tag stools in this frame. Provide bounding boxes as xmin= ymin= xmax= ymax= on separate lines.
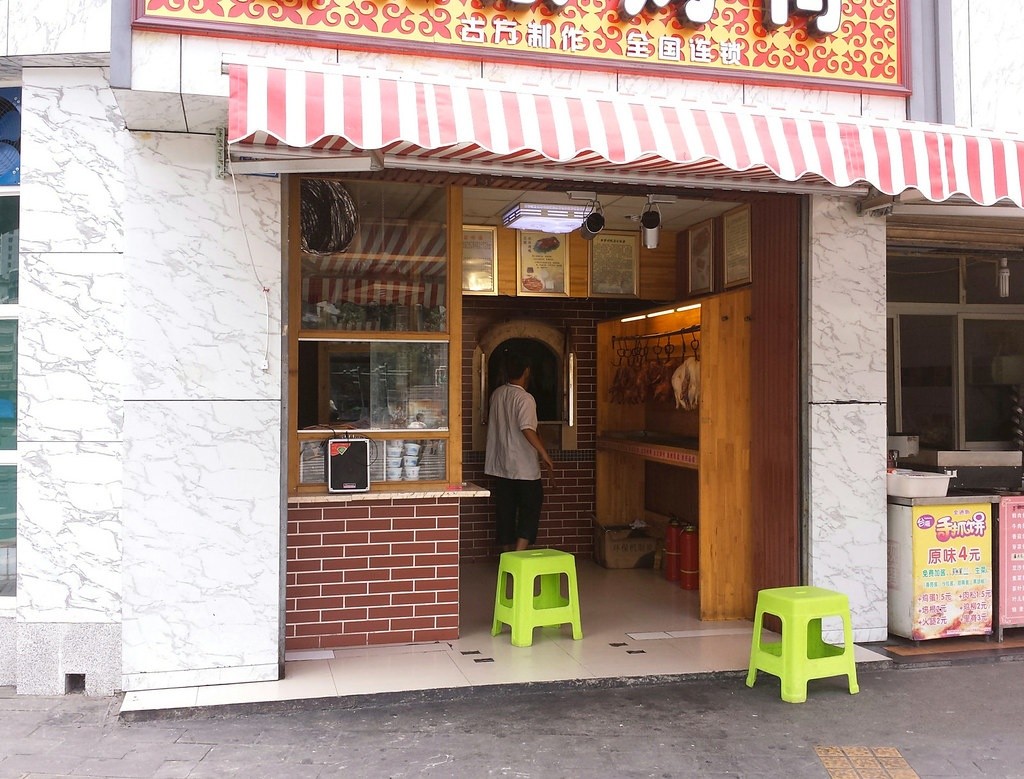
xmin=490 ymin=548 xmax=583 ymax=647
xmin=745 ymin=586 xmax=860 ymax=704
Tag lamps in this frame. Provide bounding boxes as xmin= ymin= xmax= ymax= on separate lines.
xmin=502 ymin=202 xmax=597 ymax=233
xmin=639 ymin=194 xmax=676 ymax=250
xmin=567 ymin=191 xmax=605 ymax=240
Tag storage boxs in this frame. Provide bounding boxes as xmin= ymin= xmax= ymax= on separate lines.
xmin=887 ymin=471 xmax=951 ymax=498
xmin=591 ymin=515 xmax=664 ymax=569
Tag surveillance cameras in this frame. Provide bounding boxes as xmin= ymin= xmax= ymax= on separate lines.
xmin=580 ymin=213 xmax=604 ymax=240
xmin=641 ymin=211 xmax=661 ymax=249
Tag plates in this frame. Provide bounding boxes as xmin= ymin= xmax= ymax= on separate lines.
xmin=299 ymin=443 xmax=447 ymax=484
xmin=522 ymin=275 xmax=545 ymax=292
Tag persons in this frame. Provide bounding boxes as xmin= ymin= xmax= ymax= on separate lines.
xmin=394 ymin=404 xmax=447 ymax=464
xmin=484 ymin=357 xmax=554 ymax=553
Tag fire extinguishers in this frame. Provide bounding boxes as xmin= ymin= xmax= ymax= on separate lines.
xmin=662 ymin=515 xmax=681 ymax=583
xmin=678 ymin=520 xmax=699 ymax=590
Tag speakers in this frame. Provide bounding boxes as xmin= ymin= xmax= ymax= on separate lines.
xmin=325 ymin=438 xmax=370 ymax=492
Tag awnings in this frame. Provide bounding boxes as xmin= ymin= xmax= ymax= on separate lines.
xmin=219 ymin=54 xmax=1024 ymax=211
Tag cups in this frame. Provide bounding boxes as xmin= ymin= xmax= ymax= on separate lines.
xmin=386 ymin=457 xmax=402 ymax=467
xmin=387 ymin=468 xmax=402 ymax=481
xmin=402 ymin=455 xmax=419 ymax=467
xmin=404 ymin=443 xmax=420 ymax=456
xmin=387 ymin=447 xmax=402 ymax=457
xmin=403 ymin=467 xmax=419 ymax=480
xmin=391 ymin=439 xmax=404 ymax=448
